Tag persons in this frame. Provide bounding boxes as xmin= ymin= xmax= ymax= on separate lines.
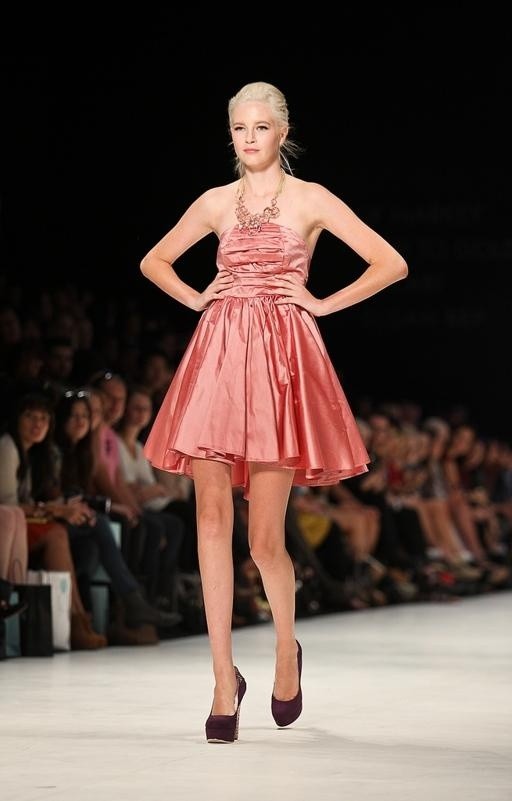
xmin=1 ymin=278 xmax=511 ymax=661
xmin=139 ymin=80 xmax=411 ymax=744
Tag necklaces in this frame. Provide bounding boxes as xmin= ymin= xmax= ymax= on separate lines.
xmin=237 ymin=168 xmax=286 ymax=239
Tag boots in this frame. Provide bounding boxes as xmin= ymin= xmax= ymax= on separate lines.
xmin=71 ymin=613 xmax=108 ymax=650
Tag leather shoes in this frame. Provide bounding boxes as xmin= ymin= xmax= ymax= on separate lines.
xmin=271 ymin=638 xmax=303 ymax=727
xmin=205 ymin=666 xmax=246 ymax=744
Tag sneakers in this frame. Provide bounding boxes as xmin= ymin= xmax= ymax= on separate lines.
xmin=144 ymin=607 xmax=183 ymax=629
